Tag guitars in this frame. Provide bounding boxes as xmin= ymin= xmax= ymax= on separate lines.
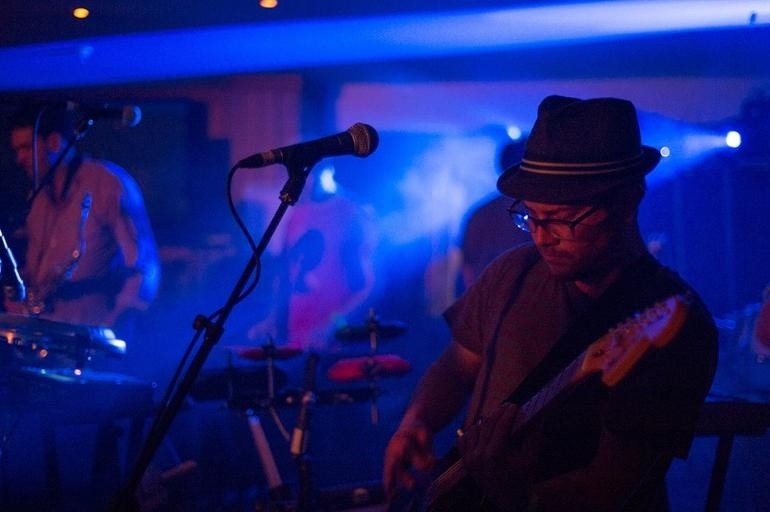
xmin=386 ymin=295 xmax=687 ymax=512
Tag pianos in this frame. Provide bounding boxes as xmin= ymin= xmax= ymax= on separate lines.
xmin=227 ymin=389 xmax=385 ymax=406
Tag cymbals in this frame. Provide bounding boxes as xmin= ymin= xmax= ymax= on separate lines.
xmin=191 ymin=366 xmax=286 ymax=402
xmin=333 ymin=319 xmax=408 ymax=344
xmin=326 ymin=355 xmax=410 ymax=379
xmin=237 ymin=346 xmax=296 ymax=362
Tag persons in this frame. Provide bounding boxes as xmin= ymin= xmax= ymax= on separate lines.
xmin=382 ymin=93 xmax=721 ymax=512
xmin=1 ymin=101 xmax=164 ymax=343
xmin=455 ymin=135 xmax=531 ymax=299
xmin=755 ymin=286 xmax=770 ymax=355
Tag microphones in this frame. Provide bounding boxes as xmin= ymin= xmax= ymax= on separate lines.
xmin=240 ymin=122 xmax=379 ymax=168
xmin=56 ymin=100 xmax=142 ymax=128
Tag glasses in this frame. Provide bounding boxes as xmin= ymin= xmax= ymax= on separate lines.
xmin=506 ymin=199 xmax=605 ymax=241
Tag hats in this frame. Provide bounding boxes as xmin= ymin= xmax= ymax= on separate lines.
xmin=497 ymin=94 xmax=660 ymax=205
